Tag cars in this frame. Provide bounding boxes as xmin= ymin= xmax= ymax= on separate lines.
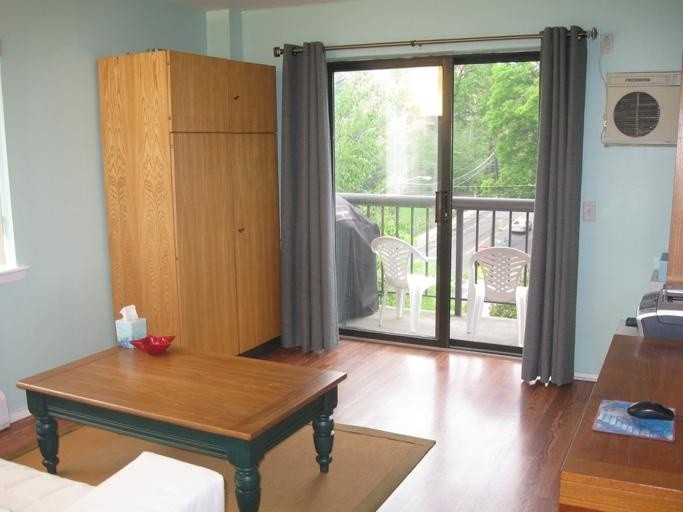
xmin=512 ymin=220 xmax=532 ymax=233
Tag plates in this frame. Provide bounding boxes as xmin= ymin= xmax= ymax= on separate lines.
xmin=128 ymin=335 xmax=176 ymax=356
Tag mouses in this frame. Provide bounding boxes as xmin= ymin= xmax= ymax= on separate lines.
xmin=627 ymin=401 xmax=674 ymax=420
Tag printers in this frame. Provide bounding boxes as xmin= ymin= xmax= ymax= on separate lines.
xmin=636 ymin=284 xmax=683 ymax=340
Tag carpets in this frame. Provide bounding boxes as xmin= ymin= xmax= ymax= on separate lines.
xmin=0 ymin=409 xmax=437 ymax=512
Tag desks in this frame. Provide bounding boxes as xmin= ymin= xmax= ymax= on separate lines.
xmin=562 ymin=332 xmax=683 ymax=508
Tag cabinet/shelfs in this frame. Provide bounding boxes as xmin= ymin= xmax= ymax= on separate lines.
xmin=98 ymin=54 xmax=277 ymax=358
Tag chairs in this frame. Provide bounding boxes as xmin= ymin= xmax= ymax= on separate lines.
xmin=368 ymin=235 xmax=439 ymax=331
xmin=463 ymin=248 xmax=532 ymax=347
